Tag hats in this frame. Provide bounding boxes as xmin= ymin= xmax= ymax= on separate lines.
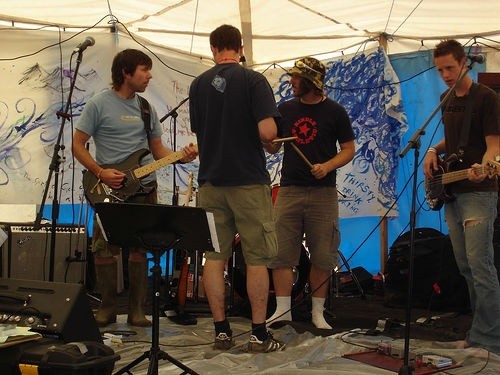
xmin=286 ymin=57 xmax=326 ymax=92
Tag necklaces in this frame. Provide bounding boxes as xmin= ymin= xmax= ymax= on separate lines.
xmin=216 ymin=57 xmax=238 ymax=63
xmin=319 ymin=95 xmax=324 ymax=102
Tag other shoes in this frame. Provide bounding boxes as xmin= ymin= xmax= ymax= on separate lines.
xmin=456 ymin=340 xmax=500 ymax=360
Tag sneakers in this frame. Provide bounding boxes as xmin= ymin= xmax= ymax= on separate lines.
xmin=247 ymin=330 xmax=287 ymax=353
xmin=215 ymin=330 xmax=236 ymax=350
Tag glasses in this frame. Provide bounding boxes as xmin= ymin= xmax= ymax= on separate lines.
xmin=295 ymin=61 xmax=320 ymax=74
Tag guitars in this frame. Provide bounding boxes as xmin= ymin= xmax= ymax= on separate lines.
xmin=81 ymin=142 xmax=199 ymax=213
xmin=424 ymin=152 xmax=500 ymax=212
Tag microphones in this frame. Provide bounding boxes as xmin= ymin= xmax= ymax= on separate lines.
xmin=467 ymin=54 xmax=486 ymax=64
xmin=71 ymin=36 xmax=96 ymax=56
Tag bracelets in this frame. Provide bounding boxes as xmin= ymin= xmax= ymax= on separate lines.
xmin=177 ymin=160 xmax=186 ymax=164
xmin=97 ymin=168 xmax=103 ymax=178
xmin=427 ymin=147 xmax=439 ymax=155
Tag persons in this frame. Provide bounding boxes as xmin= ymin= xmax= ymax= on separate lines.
xmin=266 ymin=56 xmax=356 ymax=331
xmin=70 ymin=48 xmax=197 ymax=326
xmin=189 ymin=25 xmax=287 ymax=353
xmin=423 ymin=39 xmax=500 ymax=351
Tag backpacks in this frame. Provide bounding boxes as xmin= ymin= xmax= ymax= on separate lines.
xmin=383 ymin=228 xmax=469 ymax=311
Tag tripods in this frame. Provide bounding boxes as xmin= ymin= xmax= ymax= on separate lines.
xmin=93 ymin=202 xmax=214 ymax=375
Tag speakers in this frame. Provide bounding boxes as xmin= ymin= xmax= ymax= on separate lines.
xmin=1 ymin=223 xmax=103 ymax=342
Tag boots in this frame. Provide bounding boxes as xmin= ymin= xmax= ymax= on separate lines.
xmin=94 ymin=262 xmax=117 ymax=327
xmin=127 ymin=260 xmax=152 ymax=327
